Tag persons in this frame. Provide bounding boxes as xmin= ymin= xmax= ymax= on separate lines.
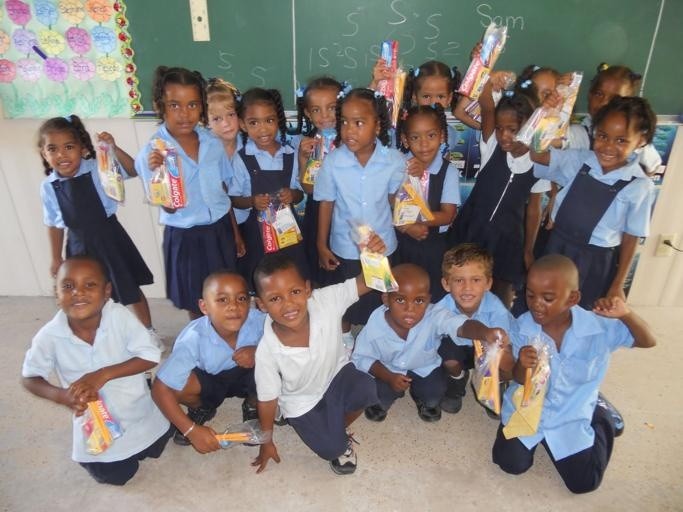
xmin=316 ymin=86 xmax=423 ymax=286
xmin=350 ymin=263 xmax=508 ymax=422
xmin=228 ymin=88 xmax=316 ymax=292
xmin=435 ymin=244 xmax=517 ymax=420
xmin=370 ymin=58 xmax=463 ymax=160
xmin=491 ymin=254 xmax=656 ymax=493
xmin=204 ymin=76 xmax=241 ymax=173
xmin=388 ymin=103 xmax=463 ymax=302
xmin=147 ymin=66 xmax=247 ymax=323
xmin=35 ymin=114 xmax=168 ymax=355
xmin=445 ymin=72 xmax=553 ymax=316
xmin=252 ymin=232 xmax=385 ymax=475
xmin=287 ymin=78 xmax=345 ymax=237
xmin=550 ymin=62 xmax=660 ymax=175
xmin=151 ymin=271 xmax=266 ymax=448
xmin=19 ymin=253 xmax=180 ymax=483
xmin=454 ymin=64 xmax=564 ymax=132
xmin=529 ymin=90 xmax=657 ymax=312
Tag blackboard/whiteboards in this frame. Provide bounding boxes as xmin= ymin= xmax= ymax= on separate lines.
xmin=115 ymin=1 xmax=683 ymax=126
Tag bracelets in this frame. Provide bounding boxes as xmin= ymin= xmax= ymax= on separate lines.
xmin=180 ymin=421 xmax=197 ymax=441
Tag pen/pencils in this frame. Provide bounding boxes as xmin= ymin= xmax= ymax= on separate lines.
xmin=97 ymin=149 xmax=107 ymax=171
xmin=490 ymin=39 xmax=504 ymax=68
xmin=389 ymin=273 xmax=399 ymax=290
xmin=393 ymin=71 xmax=405 ymax=128
xmin=87 ymin=402 xmax=113 ymax=446
xmin=519 ymin=361 xmax=540 ymax=408
xmin=402 ymin=182 xmax=434 ymax=222
xmin=213 ymin=431 xmax=253 ymax=443
xmin=473 ymin=340 xmax=501 ymax=415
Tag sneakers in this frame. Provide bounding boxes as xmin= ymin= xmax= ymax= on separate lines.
xmin=364 ymin=405 xmax=387 ymax=422
xmin=415 ymin=400 xmax=442 ymax=423
xmin=596 ymin=390 xmax=626 ymax=438
xmin=173 ymin=406 xmax=218 ymax=446
xmin=441 ymin=369 xmax=470 ymax=414
xmin=240 ymin=400 xmax=259 ymax=447
xmin=329 ymin=429 xmax=361 ymax=476
